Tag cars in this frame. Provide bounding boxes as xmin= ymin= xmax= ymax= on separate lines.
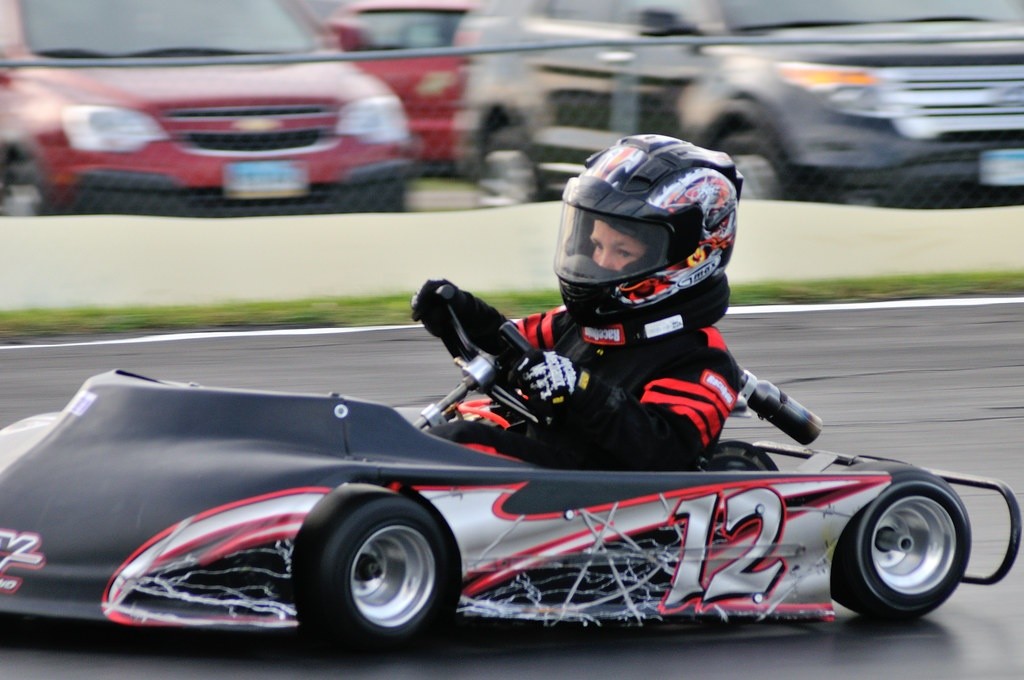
xmin=0 ymin=0 xmax=421 ymax=213
xmin=323 ymin=1 xmax=486 ymax=183
xmin=454 ymin=3 xmax=1024 ymax=204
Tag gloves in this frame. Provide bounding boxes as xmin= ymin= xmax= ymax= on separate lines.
xmin=508 ymin=348 xmax=590 ymax=422
xmin=411 ymin=276 xmax=471 ymax=337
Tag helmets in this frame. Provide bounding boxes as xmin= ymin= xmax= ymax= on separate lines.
xmin=554 ymin=134 xmax=744 ymax=327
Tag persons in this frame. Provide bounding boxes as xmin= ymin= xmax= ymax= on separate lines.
xmin=412 ymin=133 xmax=740 ymax=470
